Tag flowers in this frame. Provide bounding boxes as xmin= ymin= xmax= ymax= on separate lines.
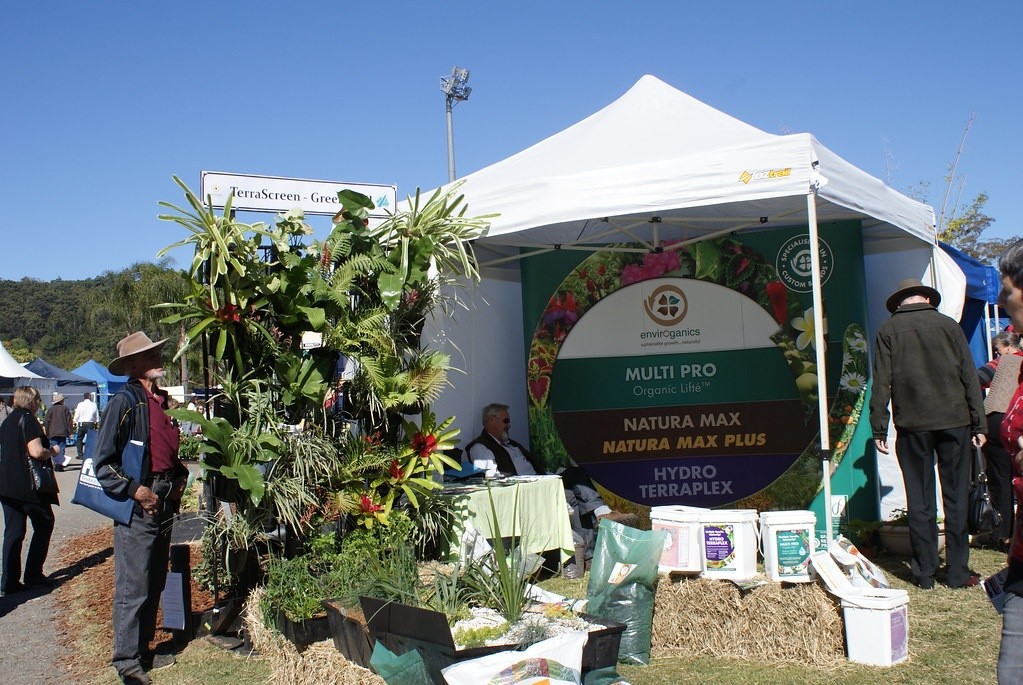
xmin=526 ymin=238 xmax=869 ymax=408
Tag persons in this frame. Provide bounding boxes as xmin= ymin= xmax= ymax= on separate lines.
xmin=465 ymin=403 xmax=639 ymax=571
xmin=0 ymin=386 xmax=61 ymax=597
xmin=73 ymin=392 xmax=100 ymax=460
xmin=169 ymin=393 xmax=213 ymax=435
xmin=44 ymin=394 xmax=73 ymax=472
xmin=978 ymin=236 xmax=1023 ymax=685
xmin=0 ymin=397 xmax=14 ymax=427
xmin=92 ymin=332 xmax=189 ymax=685
xmin=868 ymin=278 xmax=989 ymax=587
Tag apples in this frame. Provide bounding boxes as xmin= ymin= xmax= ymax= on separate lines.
xmin=777 ymin=341 xmax=818 ymax=402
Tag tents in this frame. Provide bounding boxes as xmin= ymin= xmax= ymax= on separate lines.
xmin=0 ymin=340 xmax=129 ymax=446
xmin=359 ymin=73 xmax=1009 ymax=560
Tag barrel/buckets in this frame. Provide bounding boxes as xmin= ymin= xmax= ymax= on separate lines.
xmin=649 ymin=505 xmax=711 ymax=572
xmin=699 ymin=510 xmax=760 ymax=583
xmin=758 ymin=509 xmax=817 ymax=584
xmin=841 ymin=588 xmax=910 ymax=667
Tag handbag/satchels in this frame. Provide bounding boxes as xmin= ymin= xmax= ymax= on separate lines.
xmin=27 ymin=455 xmax=61 ymax=494
xmin=68 ymin=389 xmax=142 ymax=526
xmin=966 ymin=444 xmax=1004 ymax=533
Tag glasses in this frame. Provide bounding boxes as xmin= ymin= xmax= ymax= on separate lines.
xmin=503 ymin=417 xmax=511 ymax=423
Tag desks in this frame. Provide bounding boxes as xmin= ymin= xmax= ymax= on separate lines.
xmin=440 ymin=472 xmax=575 ymax=577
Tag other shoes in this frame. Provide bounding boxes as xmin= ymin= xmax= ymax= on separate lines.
xmin=145 ymin=652 xmax=177 ymax=668
xmin=75 ymin=455 xmax=84 ymax=460
xmin=123 ymin=668 xmax=152 ymax=685
xmin=955 ymin=572 xmax=977 ymax=588
xmin=54 ymin=464 xmax=64 ymax=472
xmin=28 ymin=577 xmax=58 ymax=588
xmin=595 ymin=509 xmax=640 ymax=530
xmin=2 ymin=582 xmax=28 ymax=594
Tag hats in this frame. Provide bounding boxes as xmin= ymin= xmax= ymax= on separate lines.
xmin=887 ymin=278 xmax=942 ymax=309
xmin=51 ymin=392 xmax=69 ymax=405
xmin=106 ymin=330 xmax=168 ymax=379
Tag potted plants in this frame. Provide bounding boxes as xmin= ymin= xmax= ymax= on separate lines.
xmin=843 ymin=520 xmax=881 ymax=559
xmin=878 ymin=506 xmax=946 ymax=555
xmin=263 ymin=478 xmax=628 ymax=670
